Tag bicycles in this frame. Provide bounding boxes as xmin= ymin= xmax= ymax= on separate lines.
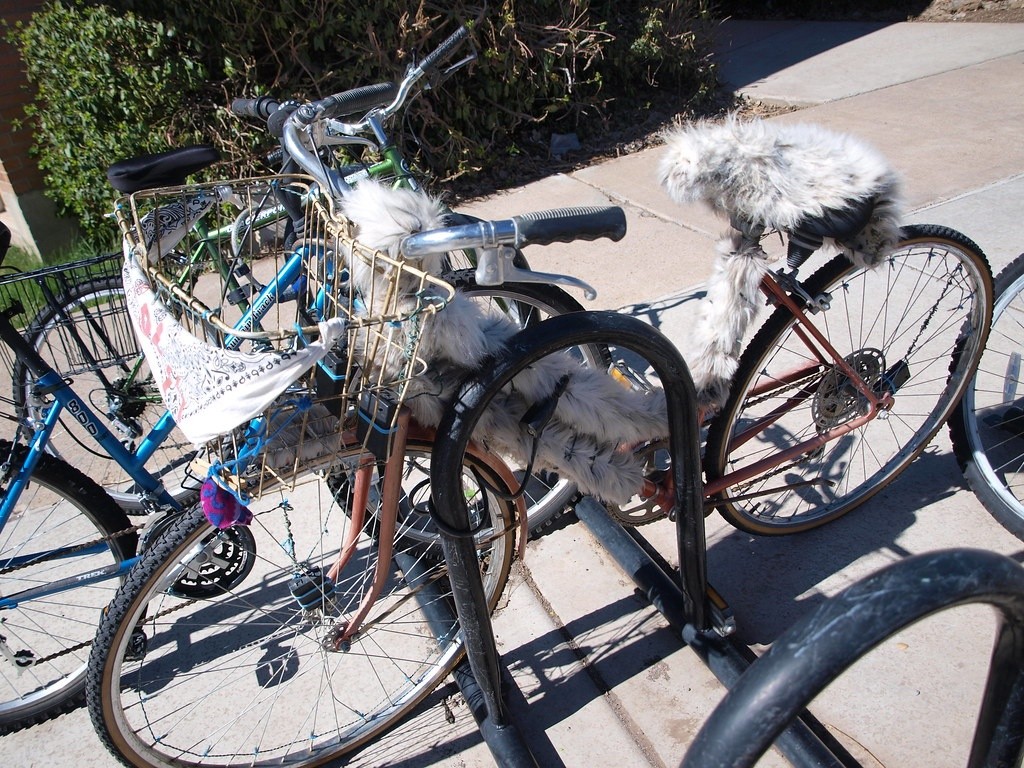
xmin=0 ymin=24 xmax=1022 ymax=767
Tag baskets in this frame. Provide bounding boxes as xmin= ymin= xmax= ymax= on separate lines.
xmin=0 ymin=252 xmax=143 ymax=377
xmin=112 ymin=176 xmax=454 ymax=505
